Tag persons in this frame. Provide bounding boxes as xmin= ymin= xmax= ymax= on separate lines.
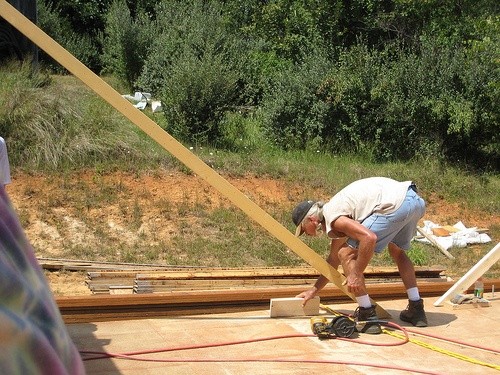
xmin=291 ymin=177 xmax=428 ymax=326
xmin=0 ymin=136 xmax=11 ymax=188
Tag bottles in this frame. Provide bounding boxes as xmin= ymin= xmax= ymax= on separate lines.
xmin=475 ymin=278 xmax=484 ymax=299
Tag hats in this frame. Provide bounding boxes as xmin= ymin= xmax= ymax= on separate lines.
xmin=292 ymin=200 xmax=317 ymax=238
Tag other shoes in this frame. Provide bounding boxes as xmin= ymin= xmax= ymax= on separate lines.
xmin=399 ymin=299 xmax=428 ymax=327
xmin=350 ymin=305 xmax=383 ymax=335
